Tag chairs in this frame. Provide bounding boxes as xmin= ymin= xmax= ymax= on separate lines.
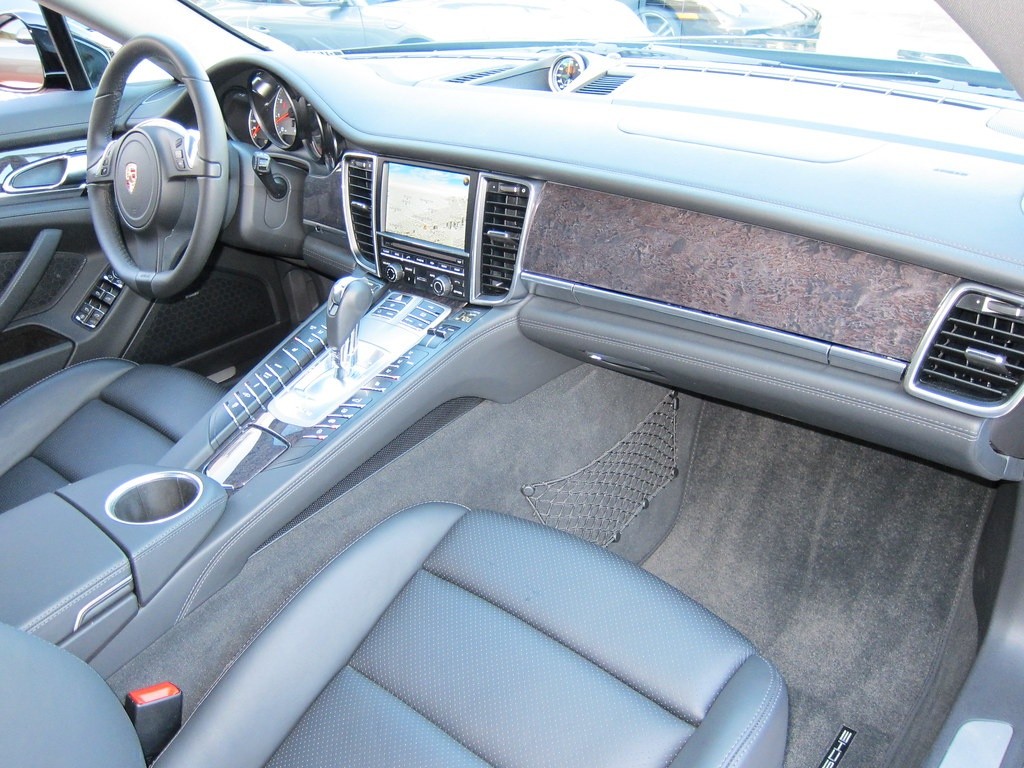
xmin=0 ymin=499 xmax=790 ymax=768
xmin=0 ymin=358 xmax=233 ymax=514
